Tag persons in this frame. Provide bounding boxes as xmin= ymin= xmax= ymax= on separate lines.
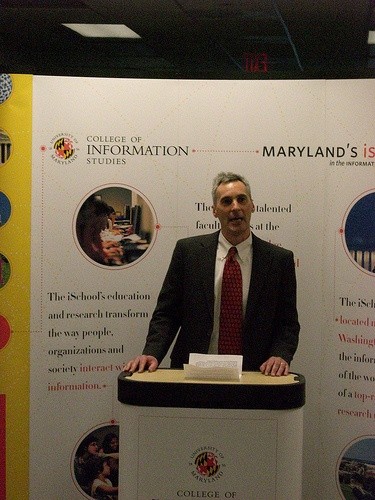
xmin=74 ymin=433 xmax=119 ymax=500
xmin=125 ymin=168 xmax=301 ymax=378
xmin=76 ymin=196 xmax=134 ymax=266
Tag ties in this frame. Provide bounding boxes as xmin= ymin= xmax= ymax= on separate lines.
xmin=218 ymin=247 xmax=244 ymax=355
